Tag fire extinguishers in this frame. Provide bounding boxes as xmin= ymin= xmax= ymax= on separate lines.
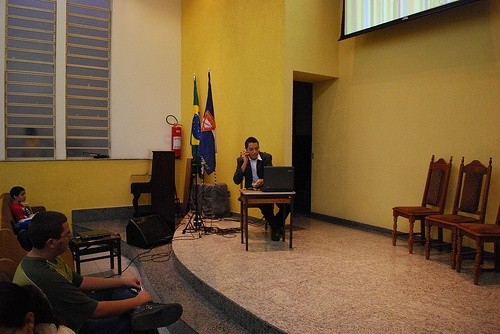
xmin=166 ymin=115 xmax=182 ymax=158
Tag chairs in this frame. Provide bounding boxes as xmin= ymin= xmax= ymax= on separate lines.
xmin=391 ymin=155 xmax=500 ymax=285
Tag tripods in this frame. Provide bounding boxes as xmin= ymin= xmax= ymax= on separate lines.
xmin=183 ymin=162 xmax=207 ymax=234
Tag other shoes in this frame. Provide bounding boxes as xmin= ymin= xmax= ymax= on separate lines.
xmin=271 ymin=227 xmax=280 ymax=241
xmin=131 ymin=303 xmax=183 ymax=331
xmin=276 ymin=212 xmax=283 ymax=233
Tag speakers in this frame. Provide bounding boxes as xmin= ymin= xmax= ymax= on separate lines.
xmin=125 ymin=215 xmax=173 ymax=248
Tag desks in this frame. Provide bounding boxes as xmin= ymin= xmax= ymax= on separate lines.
xmin=71 ymin=235 xmax=121 ymax=275
xmin=240 ymin=189 xmax=296 ymax=251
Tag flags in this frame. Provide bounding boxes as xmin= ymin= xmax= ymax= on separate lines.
xmin=198 ymin=79 xmax=216 ymax=175
xmin=190 ymin=81 xmax=202 ymax=162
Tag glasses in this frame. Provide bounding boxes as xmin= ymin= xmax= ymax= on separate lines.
xmin=60 ymin=233 xmax=73 ymax=240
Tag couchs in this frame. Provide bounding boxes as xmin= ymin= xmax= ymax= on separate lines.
xmin=0 ymin=193 xmax=75 ymax=283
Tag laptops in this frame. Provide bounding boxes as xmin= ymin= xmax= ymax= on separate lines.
xmin=260 ymin=166 xmax=294 ymax=192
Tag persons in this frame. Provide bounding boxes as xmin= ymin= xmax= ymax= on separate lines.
xmin=9 ymin=187 xmax=33 ymax=220
xmin=0 ymin=281 xmax=76 ymax=334
xmin=233 ymin=137 xmax=291 ymax=241
xmin=12 ymin=210 xmax=184 ymax=334
